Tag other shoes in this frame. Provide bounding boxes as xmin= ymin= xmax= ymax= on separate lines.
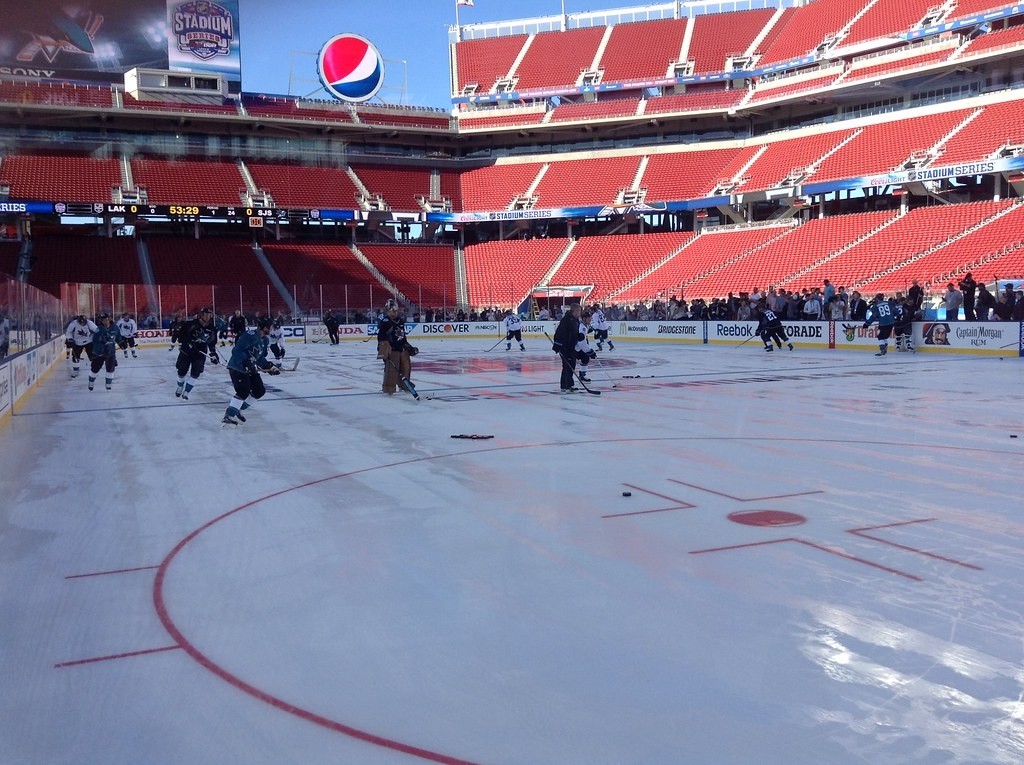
xmin=765 ymin=344 xmax=773 ymax=351
xmin=124 ymin=351 xmax=138 ymax=358
xmin=896 ymin=346 xmax=903 ymax=353
xmin=906 ymin=346 xmax=915 ymax=353
xmin=220 ymin=340 xmax=234 ymax=347
xmin=505 ymin=340 xmax=615 ymax=394
xmin=169 ymin=344 xmax=174 ymax=350
xmin=788 ymin=342 xmax=794 ymax=351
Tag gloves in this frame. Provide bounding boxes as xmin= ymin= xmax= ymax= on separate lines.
xmin=755 ymin=330 xmax=760 ymax=336
xmin=281 ymin=348 xmax=286 ymax=358
xmin=265 ymin=361 xmax=280 ymax=376
xmin=120 ymin=341 xmax=127 ymax=349
xmin=587 ymin=349 xmax=597 ymax=360
xmin=588 ymin=327 xmax=593 ymax=334
xmin=101 ymin=347 xmax=111 ymax=357
xmin=209 ymin=353 xmax=219 ymax=364
xmin=245 ymin=361 xmax=257 ymax=374
xmin=552 ymin=341 xmax=563 ymax=353
xmin=186 ymin=342 xmax=198 ymax=353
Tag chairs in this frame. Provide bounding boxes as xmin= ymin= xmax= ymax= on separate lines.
xmin=0 ymin=0 xmax=1024 ymax=324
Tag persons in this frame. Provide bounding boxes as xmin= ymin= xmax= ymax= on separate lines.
xmin=0 ymin=273 xmax=1024 ymax=401
xmin=923 ymin=322 xmax=951 ymax=345
xmin=222 ymin=315 xmax=280 ymax=428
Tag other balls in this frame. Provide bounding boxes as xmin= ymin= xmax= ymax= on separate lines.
xmin=623 ymin=492 xmax=631 ymax=496
xmin=1000 ymin=358 xmax=1003 ymax=360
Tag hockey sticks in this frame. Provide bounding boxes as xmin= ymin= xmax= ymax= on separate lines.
xmin=312 ymin=333 xmax=329 ymax=343
xmin=544 ymin=332 xmax=601 ymax=395
xmin=215 ymin=346 xmax=227 ymax=363
xmin=72 ymin=347 xmax=84 ymax=361
xmin=171 ymin=335 xmax=180 ymax=343
xmin=388 ymin=357 xmax=434 ymax=401
xmin=484 ymin=336 xmax=508 ymax=352
xmin=258 ymin=356 xmax=300 ymax=372
xmin=841 ymin=323 xmax=879 ymax=330
xmin=132 ymin=332 xmax=161 ymax=337
xmin=198 ymin=350 xmax=226 ymax=367
xmin=735 ymin=335 xmax=756 ymax=348
xmin=363 ymin=329 xmax=378 ymax=342
xmin=595 ymin=356 xmax=617 ymax=388
xmin=91 ymin=347 xmax=122 ymax=360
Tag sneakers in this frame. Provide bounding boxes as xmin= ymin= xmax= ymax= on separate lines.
xmin=175 ymin=386 xmax=189 ymax=402
xmin=66 ymin=355 xmax=112 ymax=392
xmin=220 ymin=411 xmax=246 ymax=430
xmin=875 ymin=349 xmax=888 ymax=358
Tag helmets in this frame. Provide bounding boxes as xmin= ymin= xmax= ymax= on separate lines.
xmin=200 ymin=307 xmax=213 ymax=314
xmin=757 ymin=302 xmax=765 ymax=308
xmin=258 ymin=313 xmax=282 ymax=328
xmin=385 ymin=299 xmax=399 ymax=311
